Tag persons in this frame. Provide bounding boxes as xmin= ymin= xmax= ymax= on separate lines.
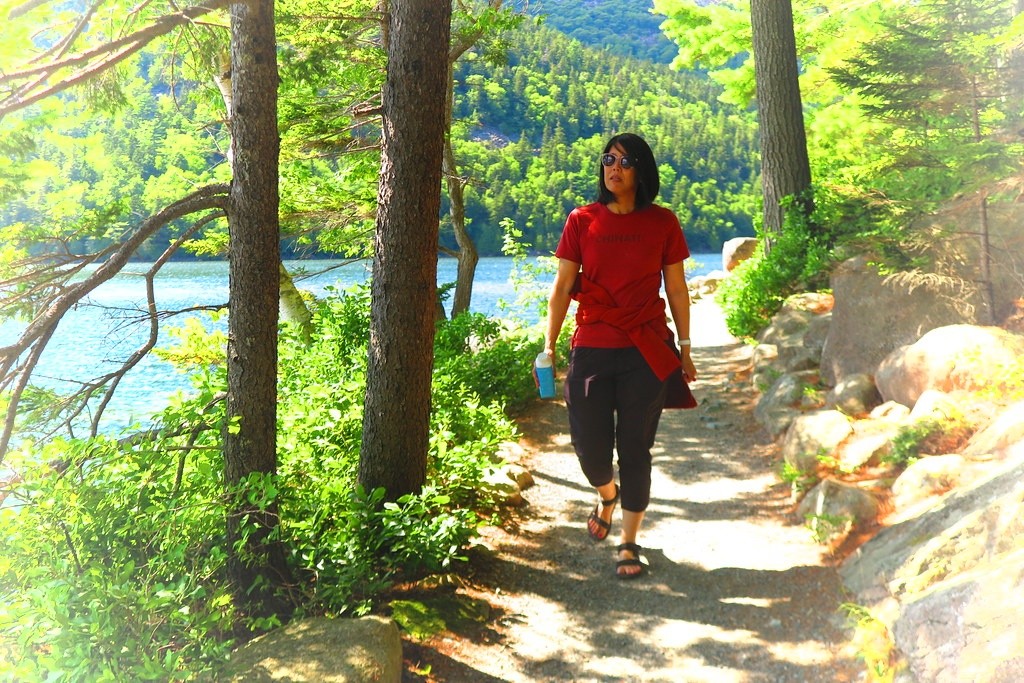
xmin=532 ymin=133 xmax=697 ymax=580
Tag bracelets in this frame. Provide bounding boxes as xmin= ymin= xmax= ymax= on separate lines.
xmin=678 ymin=340 xmax=691 ymax=345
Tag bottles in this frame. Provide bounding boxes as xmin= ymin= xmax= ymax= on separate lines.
xmin=536 ymin=353 xmax=556 ymax=400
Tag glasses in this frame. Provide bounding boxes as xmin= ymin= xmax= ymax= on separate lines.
xmin=601 ymin=153 xmax=639 ymax=169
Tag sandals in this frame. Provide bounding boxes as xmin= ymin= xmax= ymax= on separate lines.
xmin=587 ymin=483 xmax=619 ymax=541
xmin=615 ymin=543 xmax=642 ymax=580
